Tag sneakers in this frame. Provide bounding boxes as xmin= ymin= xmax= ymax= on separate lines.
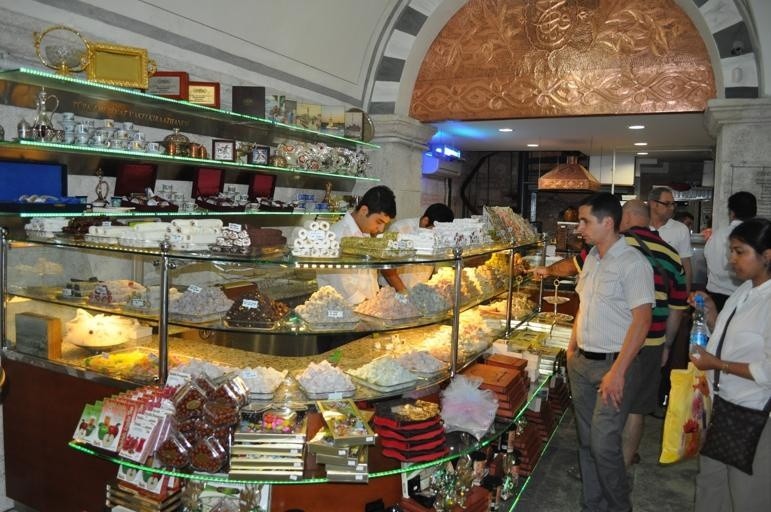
xmin=626 ymin=464 xmax=636 ymax=492
xmin=565 ymin=465 xmax=583 ymax=483
xmin=649 ymin=400 xmax=667 ymax=420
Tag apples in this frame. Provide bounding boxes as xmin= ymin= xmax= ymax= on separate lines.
xmin=78 ymin=419 xmax=145 ymax=453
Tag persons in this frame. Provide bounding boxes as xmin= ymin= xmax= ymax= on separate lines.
xmin=648 ymin=186 xmax=692 ymax=314
xmin=673 ymin=211 xmax=696 ymax=230
xmin=524 ymin=198 xmax=695 ymax=498
xmin=565 ymin=193 xmax=659 ymax=512
xmin=704 ymin=192 xmax=759 ymax=321
xmin=687 ymin=219 xmax=771 ymax=512
xmin=378 ymin=203 xmax=456 ymax=311
xmin=314 ymin=184 xmax=417 ymax=358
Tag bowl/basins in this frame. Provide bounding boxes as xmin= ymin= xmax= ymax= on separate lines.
xmin=68 ymin=335 xmax=131 ymax=353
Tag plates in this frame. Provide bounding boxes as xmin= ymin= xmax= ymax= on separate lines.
xmin=540 ymin=296 xmax=571 ymax=304
xmin=539 ymin=311 xmax=574 ymax=321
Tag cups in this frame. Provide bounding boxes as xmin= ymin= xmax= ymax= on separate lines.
xmin=76 ymin=195 xmax=89 ymax=204
xmin=295 ymin=193 xmax=330 ymax=213
xmin=124 ymin=183 xmax=291 ymax=215
xmin=57 ymin=109 xmax=161 ymax=154
xmin=16 ymin=118 xmax=65 ymax=144
xmin=111 ymin=196 xmax=122 ymax=208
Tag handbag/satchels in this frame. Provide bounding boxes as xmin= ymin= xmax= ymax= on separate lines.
xmin=698 ymin=393 xmax=770 ymax=477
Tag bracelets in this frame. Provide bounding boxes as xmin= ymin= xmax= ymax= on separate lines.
xmin=722 ymin=359 xmax=729 ymax=374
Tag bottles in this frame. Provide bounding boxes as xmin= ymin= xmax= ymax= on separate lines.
xmin=428 ymin=425 xmax=522 ymax=512
xmin=688 ymin=292 xmax=708 ymax=357
xmin=270 ymin=150 xmax=288 ymax=167
xmin=163 ymin=127 xmax=208 ymax=160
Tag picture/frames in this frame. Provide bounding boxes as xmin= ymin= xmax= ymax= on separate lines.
xmin=86 ymin=43 xmax=155 ymax=89
xmin=212 ymin=140 xmax=236 ymax=162
xmin=247 ymin=146 xmax=270 ymax=166
xmin=141 ymin=72 xmax=188 ymax=100
xmin=189 ymin=81 xmax=220 ymax=109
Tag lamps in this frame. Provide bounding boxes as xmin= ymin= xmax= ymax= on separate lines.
xmin=432 ymin=128 xmax=461 ymax=160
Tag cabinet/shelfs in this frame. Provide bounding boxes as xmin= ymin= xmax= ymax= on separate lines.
xmin=0 ymin=234 xmax=570 ymax=512
xmin=0 ymin=67 xmax=378 ymax=217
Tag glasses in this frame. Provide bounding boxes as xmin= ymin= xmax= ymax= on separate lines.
xmin=654 ymin=199 xmax=678 ymax=209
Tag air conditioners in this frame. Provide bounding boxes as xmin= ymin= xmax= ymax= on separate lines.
xmin=422 ymin=152 xmax=462 ymax=178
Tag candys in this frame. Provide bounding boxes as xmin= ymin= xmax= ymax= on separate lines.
xmin=157 ymin=373 xmax=378 ymax=484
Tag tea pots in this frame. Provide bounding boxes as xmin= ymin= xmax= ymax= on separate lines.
xmin=32 ymin=86 xmax=59 ymax=142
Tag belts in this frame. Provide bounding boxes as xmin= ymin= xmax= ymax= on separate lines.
xmin=575 ymin=343 xmax=621 ymax=362
